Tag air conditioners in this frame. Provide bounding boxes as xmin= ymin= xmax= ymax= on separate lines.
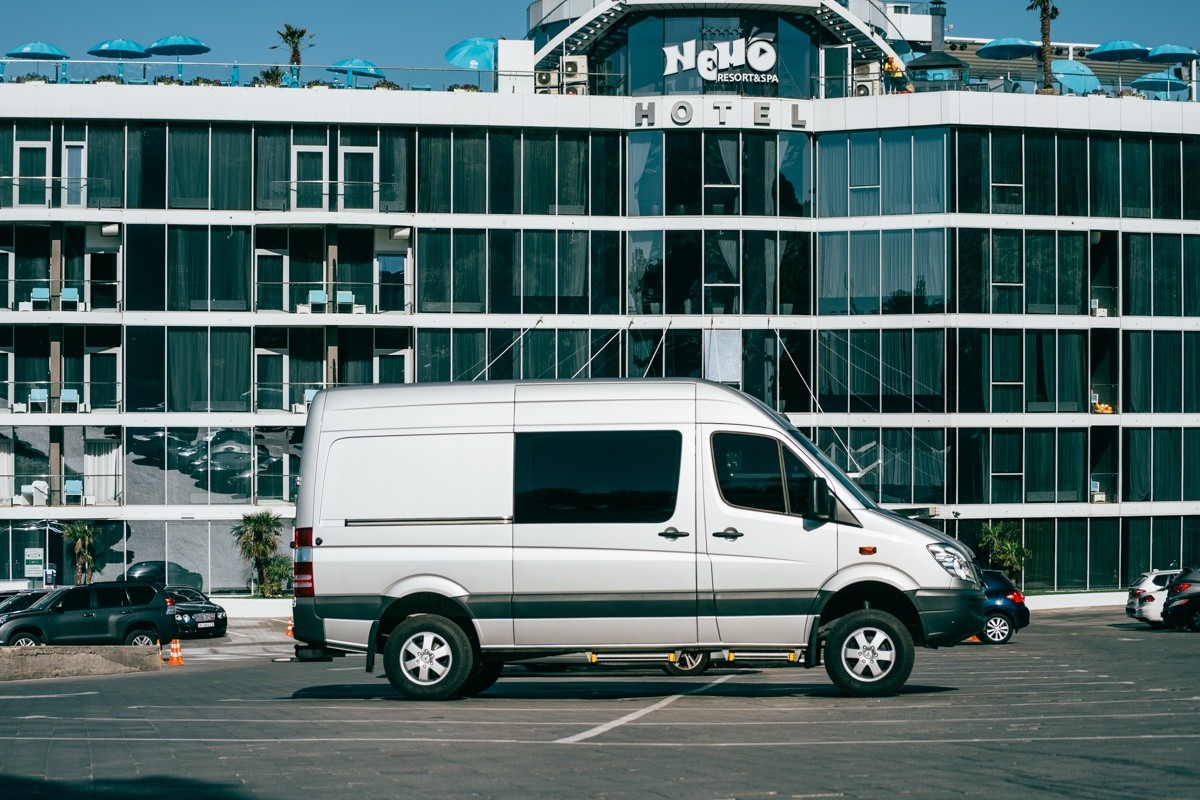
xmin=852 ymin=60 xmax=883 ymax=97
xmin=536 ymin=55 xmax=589 ymax=95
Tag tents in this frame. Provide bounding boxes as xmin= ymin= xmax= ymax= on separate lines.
xmin=907 ymin=50 xmax=969 ymax=91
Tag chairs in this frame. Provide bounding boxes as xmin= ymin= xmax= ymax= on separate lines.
xmin=21 ymin=485 xmax=34 ymax=505
xmin=59 ymin=287 xmax=79 ymax=312
xmin=308 ymin=290 xmax=328 ymax=314
xmin=60 ymin=388 xmax=80 ymax=414
xmin=1097 ymin=308 xmax=1107 ymax=317
xmin=1092 ymin=394 xmax=1099 ymax=403
xmin=28 ymin=388 xmax=48 ymax=414
xmin=335 ymin=290 xmax=355 ymax=313
xmin=64 ymin=480 xmax=83 ymax=506
xmin=1091 ymin=299 xmax=1099 ymax=316
xmin=1091 ymin=481 xmax=1100 ymax=502
xmin=31 ymin=287 xmax=50 ymax=313
xmin=304 ymin=389 xmax=320 ymax=412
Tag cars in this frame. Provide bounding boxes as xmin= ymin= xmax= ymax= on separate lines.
xmin=133 ymin=427 xmax=301 ymax=495
xmin=116 ymin=561 xmax=202 ymax=592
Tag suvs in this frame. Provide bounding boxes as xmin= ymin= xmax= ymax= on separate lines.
xmin=0 ymin=580 xmax=227 ymax=647
xmin=1126 ymin=568 xmax=1200 ymax=632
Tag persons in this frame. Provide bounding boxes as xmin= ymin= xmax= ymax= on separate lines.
xmin=885 ymin=54 xmax=904 ymax=94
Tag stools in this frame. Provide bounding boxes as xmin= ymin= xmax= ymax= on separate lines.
xmin=79 ymin=302 xmax=85 ymax=311
xmin=292 ymin=403 xmax=305 ymax=414
xmin=79 ymin=403 xmax=91 ymax=413
xmin=12 ymin=496 xmax=22 ymax=506
xmin=1094 ymin=494 xmax=1105 ymax=502
xmin=296 ymin=304 xmax=309 ymax=313
xmin=354 ymin=304 xmax=366 ymax=314
xmin=13 ymin=402 xmax=27 ymax=413
xmin=83 ymin=496 xmax=96 ymax=506
xmin=19 ymin=302 xmax=30 ymax=312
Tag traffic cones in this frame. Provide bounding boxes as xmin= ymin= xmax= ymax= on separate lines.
xmin=168 ymin=639 xmax=184 ymax=665
xmin=157 ymin=639 xmax=164 ymax=664
xmin=285 ymin=617 xmax=294 ymax=637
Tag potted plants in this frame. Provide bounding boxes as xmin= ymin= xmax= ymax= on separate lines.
xmin=17 ymin=66 xmax=480 ymax=92
xmin=1086 ymin=87 xmax=1145 ymax=100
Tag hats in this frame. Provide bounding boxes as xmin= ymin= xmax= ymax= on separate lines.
xmin=888 ymin=55 xmax=893 ymax=58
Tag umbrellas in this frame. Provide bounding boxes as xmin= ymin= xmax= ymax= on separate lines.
xmin=444 ymin=37 xmax=499 ymax=90
xmin=977 ymin=38 xmax=1041 ymax=80
xmin=1137 ymin=43 xmax=1200 ymax=99
xmin=284 ymin=70 xmax=290 ymax=87
xmin=291 ymin=62 xmax=299 ymax=88
xmin=0 ymin=57 xmax=6 ymax=83
xmin=231 ymin=59 xmax=240 ymax=87
xmin=86 ymin=38 xmax=152 ymax=84
xmin=5 ymin=40 xmax=71 ymax=75
xmin=60 ymin=57 xmax=68 ymax=84
xmin=333 ymin=72 xmax=339 ymax=88
xmin=1050 ymin=59 xmax=1101 ymax=96
xmin=326 ymin=58 xmax=386 ymax=86
xmin=1086 ymin=40 xmax=1152 ymax=91
xmin=1130 ymin=73 xmax=1188 ymax=103
xmin=144 ymin=34 xmax=211 ymax=80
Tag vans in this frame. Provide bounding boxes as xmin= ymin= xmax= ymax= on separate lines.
xmin=292 ymin=376 xmax=1030 ymax=699
